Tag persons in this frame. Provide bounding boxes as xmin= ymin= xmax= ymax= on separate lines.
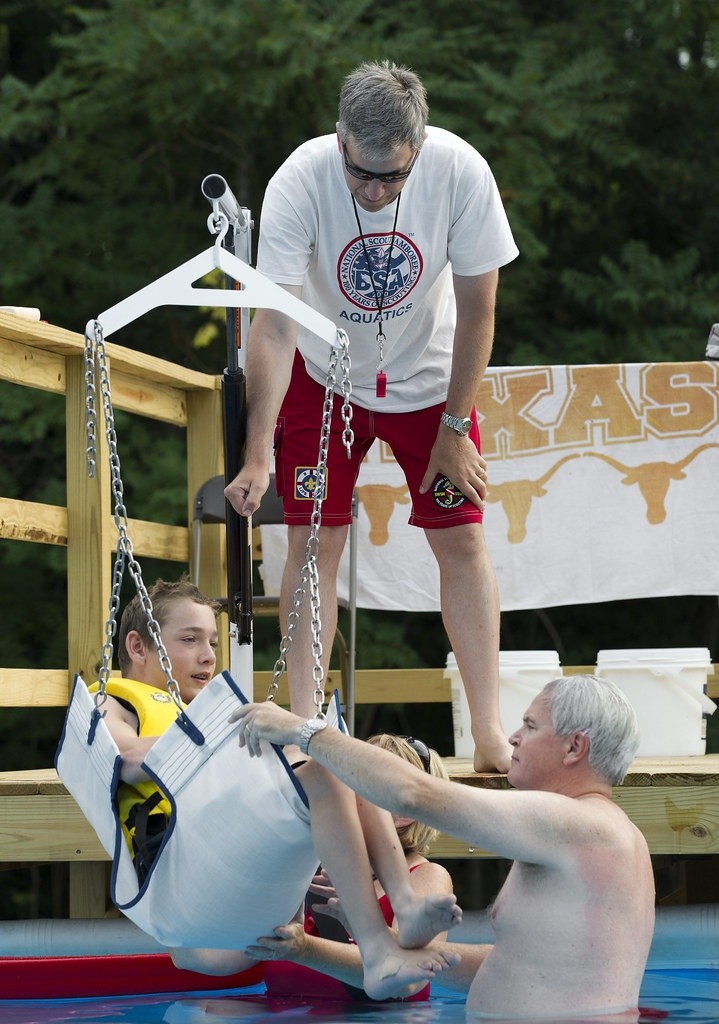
xmin=230 ymin=673 xmax=657 ymax=1024
xmin=88 ymin=575 xmax=463 ymax=1024
xmin=221 ymin=58 xmax=521 ymax=777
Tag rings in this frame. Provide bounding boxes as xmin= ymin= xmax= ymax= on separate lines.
xmin=268 ymin=952 xmax=275 ymax=960
xmin=478 ymin=468 xmax=486 ymax=477
xmin=246 ymin=725 xmax=251 ymax=732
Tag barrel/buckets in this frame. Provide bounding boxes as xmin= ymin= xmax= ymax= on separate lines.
xmin=442 ymin=651 xmax=563 ymax=758
xmin=596 ymin=647 xmax=717 ymax=756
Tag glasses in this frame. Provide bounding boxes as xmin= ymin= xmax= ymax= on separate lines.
xmin=397 ymin=732 xmax=431 ymax=775
xmin=341 ymin=142 xmax=419 ymax=183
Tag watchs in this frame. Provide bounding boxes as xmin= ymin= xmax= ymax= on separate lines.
xmin=300 ymin=718 xmax=327 ymax=756
xmin=439 ymin=412 xmax=473 ymax=436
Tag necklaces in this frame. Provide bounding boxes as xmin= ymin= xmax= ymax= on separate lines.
xmin=371 ymin=872 xmax=376 ymax=882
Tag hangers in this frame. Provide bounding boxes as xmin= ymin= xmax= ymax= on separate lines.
xmin=86 ymin=210 xmax=352 ymax=351
